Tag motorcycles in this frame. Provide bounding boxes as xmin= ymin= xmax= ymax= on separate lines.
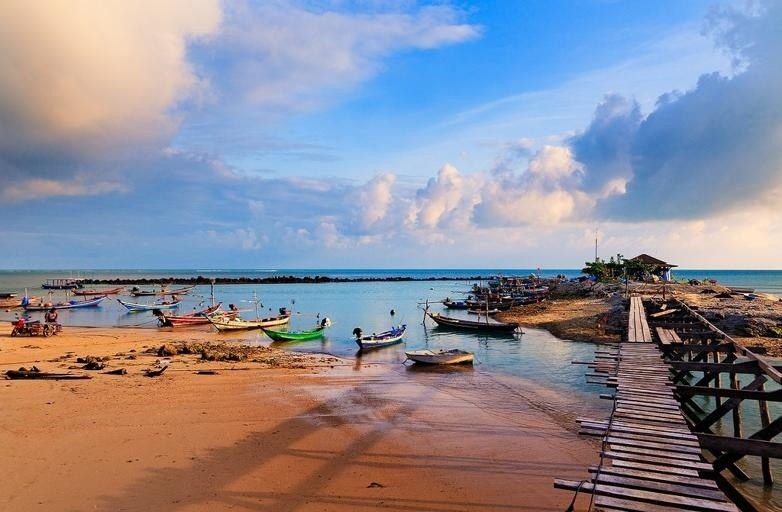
xmin=10 ymin=312 xmax=40 ymax=337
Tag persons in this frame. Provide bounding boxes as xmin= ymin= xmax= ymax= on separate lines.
xmin=22 ymin=296 xmax=28 ymax=308
xmin=44 ymin=307 xmax=58 ymax=335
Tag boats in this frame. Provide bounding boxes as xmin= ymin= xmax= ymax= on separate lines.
xmin=352 ymin=324 xmax=406 ymax=349
xmin=425 ymin=311 xmax=521 ymax=334
xmin=404 ymin=362 xmax=474 ymax=376
xmin=430 ymin=324 xmax=522 ymax=346
xmin=441 ymin=273 xmax=570 ymax=315
xmin=743 ymin=292 xmax=759 ymax=301
xmin=258 ymin=317 xmax=333 ymax=341
xmin=0 ymin=277 xmax=295 ymax=332
xmin=405 ymin=348 xmax=474 ymax=365
xmin=353 ymin=339 xmax=404 ymax=361
xmin=266 ymin=336 xmax=328 ymax=353
